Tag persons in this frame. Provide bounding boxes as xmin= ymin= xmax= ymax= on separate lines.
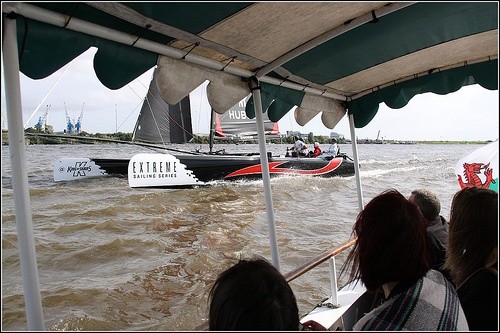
xmin=408 ymin=189 xmax=456 ymax=290
xmin=325 ymin=137 xmax=338 ymax=158
xmin=207 ymin=252 xmax=300 ymax=331
xmin=290 ymin=136 xmax=307 ymax=153
xmin=311 ymin=142 xmax=321 ymax=158
xmin=302 ymin=187 xmax=470 ymax=331
xmin=337 ymin=144 xmax=340 ymax=155
xmin=298 ymin=137 xmax=310 ymax=155
xmin=441 ymin=186 xmax=498 ymax=331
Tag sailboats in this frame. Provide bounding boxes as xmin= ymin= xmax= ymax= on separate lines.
xmin=53 ymin=69 xmax=361 ymax=190
